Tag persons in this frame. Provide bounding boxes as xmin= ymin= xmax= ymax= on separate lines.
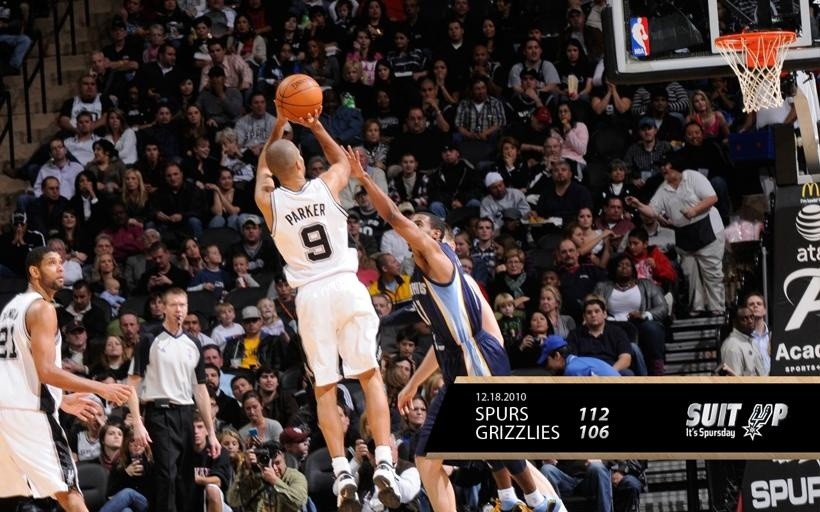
xmin=2 ymin=1 xmax=819 ymax=511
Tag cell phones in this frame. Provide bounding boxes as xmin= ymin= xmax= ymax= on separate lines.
xmin=249 ymin=428 xmax=259 ymax=440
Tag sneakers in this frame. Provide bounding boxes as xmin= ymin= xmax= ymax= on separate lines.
xmin=336 ymin=473 xmax=362 ymax=512
xmin=532 ymin=494 xmax=563 ymax=512
xmin=490 ymin=497 xmax=532 ymax=512
xmin=371 ymin=462 xmax=404 ymax=510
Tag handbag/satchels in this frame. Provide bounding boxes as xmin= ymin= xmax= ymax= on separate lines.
xmin=672 ymin=212 xmax=718 ymax=254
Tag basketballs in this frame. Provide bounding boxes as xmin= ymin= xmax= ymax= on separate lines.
xmin=274 ymin=74 xmax=322 ymax=121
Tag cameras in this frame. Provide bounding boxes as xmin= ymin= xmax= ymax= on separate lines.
xmin=363 ymin=435 xmax=376 ymax=456
xmin=250 ymin=447 xmax=270 ymax=475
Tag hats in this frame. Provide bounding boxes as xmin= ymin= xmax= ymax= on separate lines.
xmin=206 ymin=65 xmax=227 ymax=77
xmin=240 ymin=214 xmax=260 ymax=227
xmin=396 ymin=200 xmax=416 ymax=214
xmin=566 ymin=6 xmax=586 ymax=17
xmin=519 ymin=67 xmax=538 ymax=79
xmin=352 ymin=183 xmax=367 ymax=199
xmin=651 ymin=150 xmax=678 ymax=166
xmin=8 ymin=209 xmax=28 ymax=226
xmin=536 ymin=333 xmax=569 ymax=367
xmin=66 ymin=319 xmax=86 ymax=331
xmin=239 ymin=304 xmax=263 ymax=322
xmin=278 ymin=425 xmax=310 ymax=443
xmin=484 ymin=171 xmax=505 ymax=189
xmin=637 ymin=117 xmax=659 ymax=128
